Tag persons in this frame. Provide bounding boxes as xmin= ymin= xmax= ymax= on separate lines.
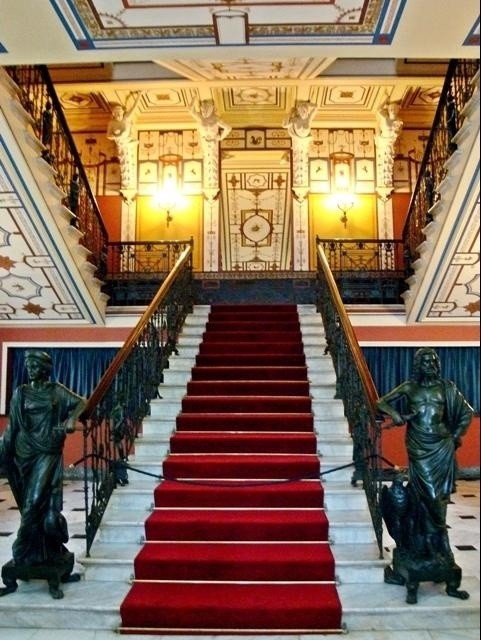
xmin=375 ymin=89 xmax=406 ymax=138
xmin=187 ymin=88 xmax=232 ymax=141
xmin=281 ymin=99 xmax=321 ymax=139
xmin=1 ymin=349 xmax=89 ymax=601
xmin=107 ymin=89 xmax=147 ymax=143
xmin=375 ymin=347 xmax=476 ymax=605
